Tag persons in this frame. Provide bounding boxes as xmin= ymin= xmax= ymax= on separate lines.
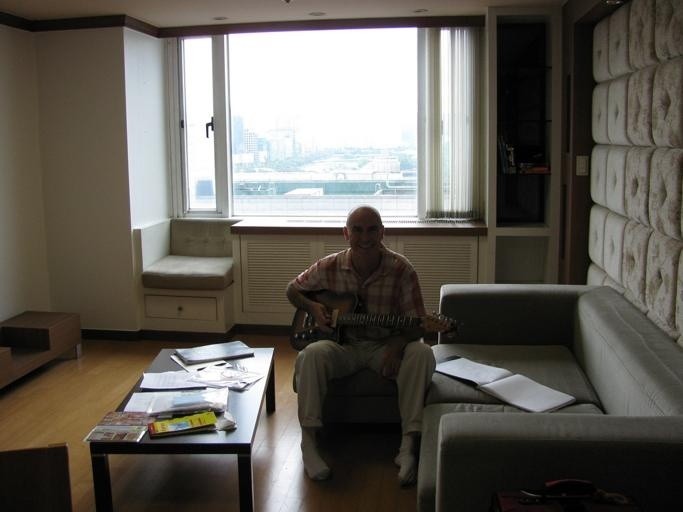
xmin=285 ymin=206 xmax=436 ymax=487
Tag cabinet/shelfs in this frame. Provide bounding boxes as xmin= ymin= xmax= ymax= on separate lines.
xmin=0 ymin=310 xmax=83 ymax=392
xmin=483 ymin=7 xmax=562 ymax=284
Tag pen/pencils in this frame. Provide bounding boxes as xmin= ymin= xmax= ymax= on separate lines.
xmin=197 ymin=363 xmax=227 ymax=371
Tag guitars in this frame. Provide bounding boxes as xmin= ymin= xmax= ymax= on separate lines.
xmin=289 ymin=289 xmax=460 ymax=351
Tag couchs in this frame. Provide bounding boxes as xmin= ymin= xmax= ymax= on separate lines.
xmin=415 ymin=284 xmax=682 ymax=511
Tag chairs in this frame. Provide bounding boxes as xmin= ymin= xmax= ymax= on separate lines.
xmin=134 ymin=216 xmax=237 ymax=338
xmin=288 ymin=303 xmax=426 ymax=429
xmin=0 ymin=441 xmax=74 ymax=510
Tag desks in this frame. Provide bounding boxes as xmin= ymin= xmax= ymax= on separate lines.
xmin=84 ymin=347 xmax=278 ymax=512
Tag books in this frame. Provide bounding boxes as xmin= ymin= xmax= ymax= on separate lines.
xmin=434 ymin=350 xmax=576 ymax=412
xmin=84 ymin=340 xmax=253 ymax=448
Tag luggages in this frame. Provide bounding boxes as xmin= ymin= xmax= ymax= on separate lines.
xmin=495 ymin=477 xmax=635 ymax=512
